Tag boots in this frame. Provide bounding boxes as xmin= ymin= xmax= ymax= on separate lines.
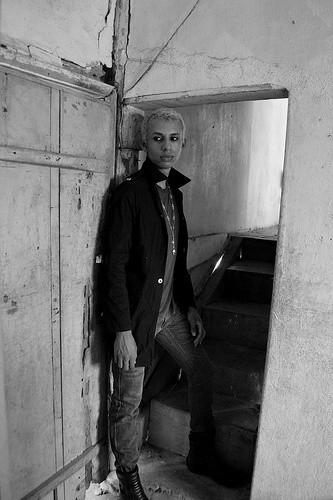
xmin=186 ymin=430 xmax=253 ymax=489
xmin=116 ymin=465 xmax=151 ymax=500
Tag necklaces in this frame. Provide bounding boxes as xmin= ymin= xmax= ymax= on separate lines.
xmin=151 ymin=181 xmax=176 ymax=256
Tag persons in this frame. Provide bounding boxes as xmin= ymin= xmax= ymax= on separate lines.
xmin=92 ymin=107 xmax=240 ymax=500
